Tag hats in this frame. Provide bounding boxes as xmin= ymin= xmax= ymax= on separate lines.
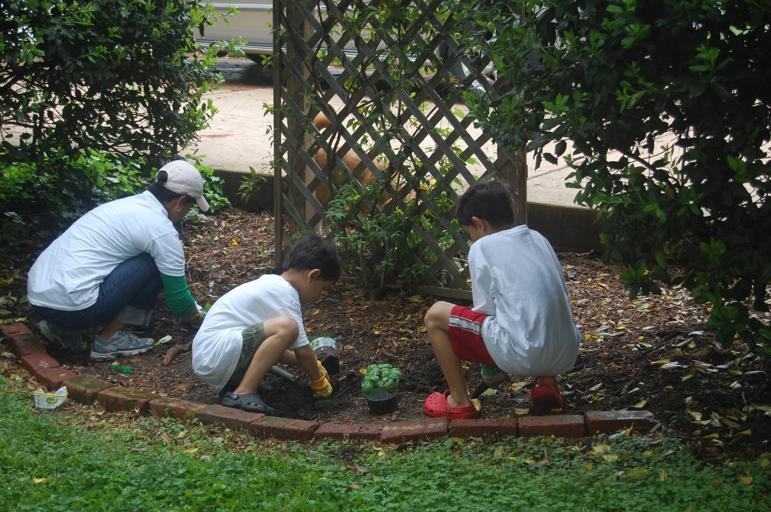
xmin=154 ymin=159 xmax=210 ymax=212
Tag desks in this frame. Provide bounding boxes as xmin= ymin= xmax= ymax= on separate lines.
xmin=241 ymin=46 xmax=287 ymax=68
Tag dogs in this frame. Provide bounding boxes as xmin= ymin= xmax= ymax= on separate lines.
xmin=301 ymin=114 xmax=419 ymax=236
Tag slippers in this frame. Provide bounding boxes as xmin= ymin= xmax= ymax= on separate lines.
xmin=220 ymin=391 xmax=279 ymax=417
xmin=530 ymin=378 xmax=564 ymax=412
xmin=422 ymin=389 xmax=476 ymax=419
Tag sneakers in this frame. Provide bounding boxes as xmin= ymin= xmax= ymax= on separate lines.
xmin=89 ymin=330 xmax=155 ymax=361
xmin=37 ymin=320 xmax=88 ymax=353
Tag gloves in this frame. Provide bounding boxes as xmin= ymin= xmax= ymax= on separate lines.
xmin=480 ymin=363 xmax=506 ymax=391
xmin=316 ymin=360 xmax=330 ymax=383
xmin=309 ymin=371 xmax=332 ymax=398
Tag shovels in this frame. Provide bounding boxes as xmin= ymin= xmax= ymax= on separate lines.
xmin=469 ymin=381 xmax=492 ymax=398
xmin=164 ymin=338 xmax=191 ymax=366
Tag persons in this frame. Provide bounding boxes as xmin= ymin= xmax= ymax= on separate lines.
xmin=191 ymin=233 xmax=341 ymax=418
xmin=27 ymin=161 xmax=209 ymax=361
xmin=424 ymin=180 xmax=581 ymax=420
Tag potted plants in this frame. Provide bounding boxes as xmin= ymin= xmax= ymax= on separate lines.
xmin=361 ymin=363 xmax=400 ymax=413
